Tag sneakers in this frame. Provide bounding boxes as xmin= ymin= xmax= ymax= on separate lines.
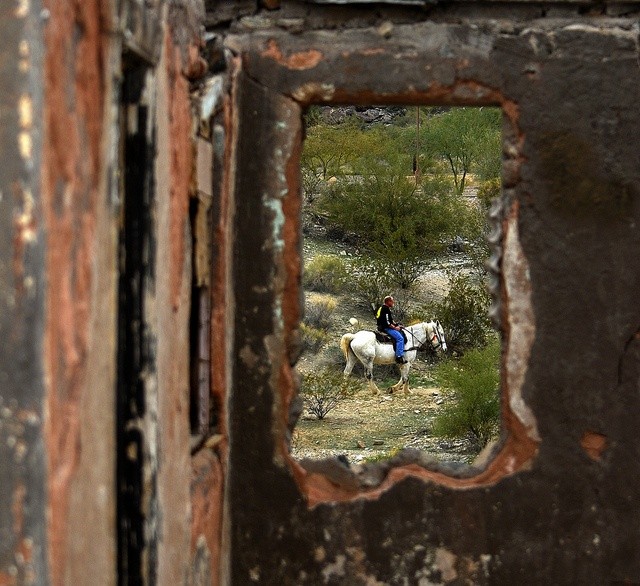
xmin=395 ymin=356 xmax=408 ymax=363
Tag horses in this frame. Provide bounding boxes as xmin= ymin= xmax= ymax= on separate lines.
xmin=340 ymin=319 xmax=448 ymax=396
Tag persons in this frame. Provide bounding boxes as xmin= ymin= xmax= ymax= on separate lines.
xmin=376 ymin=296 xmax=408 ymax=364
